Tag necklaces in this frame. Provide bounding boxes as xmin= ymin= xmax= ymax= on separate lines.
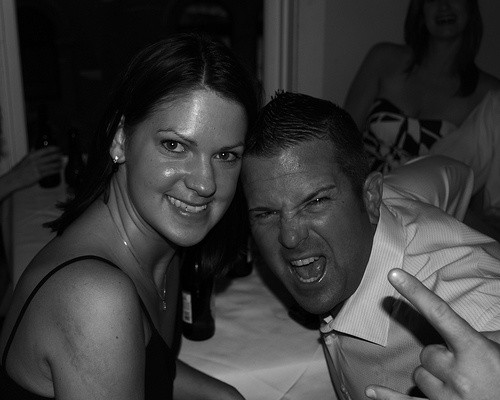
xmin=111 ymin=214 xmax=167 ymax=311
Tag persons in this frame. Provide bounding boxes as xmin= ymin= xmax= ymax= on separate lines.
xmin=345 ymin=1 xmax=500 ymax=241
xmin=0 ymin=34 xmax=268 ymax=400
xmin=0 ymin=108 xmax=63 ymax=306
xmin=177 ymin=92 xmax=500 ymax=400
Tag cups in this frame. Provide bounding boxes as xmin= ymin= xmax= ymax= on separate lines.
xmin=33 ymin=126 xmax=61 ymax=189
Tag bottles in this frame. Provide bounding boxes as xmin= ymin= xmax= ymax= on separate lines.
xmin=226 ymin=181 xmax=253 ymax=278
xmin=176 ymin=243 xmax=216 ymax=342
